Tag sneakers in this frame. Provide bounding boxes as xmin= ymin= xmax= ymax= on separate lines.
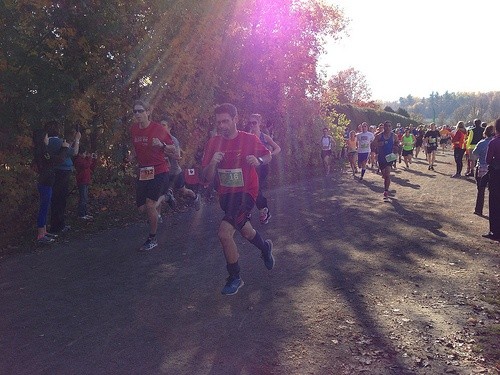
xmin=193 ymin=193 xmax=201 ymax=211
xmin=262 ymin=239 xmax=275 ymax=270
xmin=138 ymin=237 xmax=160 ymax=251
xmin=221 ymin=275 xmax=245 ymax=296
xmin=259 ymin=207 xmax=271 ymax=226
xmin=323 ymin=150 xmax=475 ymax=199
xmin=44 ymin=233 xmax=58 ymax=238
xmin=35 ymin=236 xmax=55 ymax=243
xmin=147 ymin=216 xmax=163 ymax=224
xmin=166 ymin=189 xmax=176 ymax=209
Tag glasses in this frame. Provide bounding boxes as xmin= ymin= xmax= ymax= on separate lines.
xmin=133 ymin=110 xmax=146 ymax=113
xmin=248 ymin=121 xmax=258 ymax=125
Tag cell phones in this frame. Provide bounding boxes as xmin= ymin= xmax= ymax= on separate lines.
xmin=63 ymin=137 xmax=67 ymax=143
xmin=75 ymin=124 xmax=79 ymax=133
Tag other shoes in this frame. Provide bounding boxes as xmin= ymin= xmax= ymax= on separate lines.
xmin=62 ymin=223 xmax=72 ymax=229
xmin=50 ymin=226 xmax=68 ymax=233
xmin=482 ymin=233 xmax=493 ymax=238
xmin=85 ymin=215 xmax=93 ymax=219
xmin=490 ymin=236 xmax=500 ymax=240
xmin=80 ymin=216 xmax=93 ymax=223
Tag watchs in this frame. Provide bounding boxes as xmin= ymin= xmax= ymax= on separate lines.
xmin=161 ymin=141 xmax=167 ymax=150
xmin=257 ymin=156 xmax=263 ymax=167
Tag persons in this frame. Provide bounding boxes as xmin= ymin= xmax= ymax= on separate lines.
xmin=156 ymin=114 xmax=200 ymax=213
xmin=480 ymin=118 xmax=500 ymax=242
xmin=340 ymin=118 xmax=486 ymax=179
xmin=123 ymin=99 xmax=179 ymax=253
xmin=43 ymin=120 xmax=83 ymax=234
xmin=32 ymin=129 xmax=72 ymax=247
xmin=73 ymin=142 xmax=99 ymax=224
xmin=318 ymin=127 xmax=336 ymax=176
xmin=244 ymin=112 xmax=280 ymax=223
xmin=471 ymin=125 xmax=495 ymax=215
xmin=197 ymin=103 xmax=274 ymax=297
xmin=371 ymin=120 xmax=401 ymax=199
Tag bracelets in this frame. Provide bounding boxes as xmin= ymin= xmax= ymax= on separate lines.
xmin=74 ymin=141 xmax=80 ymax=144
